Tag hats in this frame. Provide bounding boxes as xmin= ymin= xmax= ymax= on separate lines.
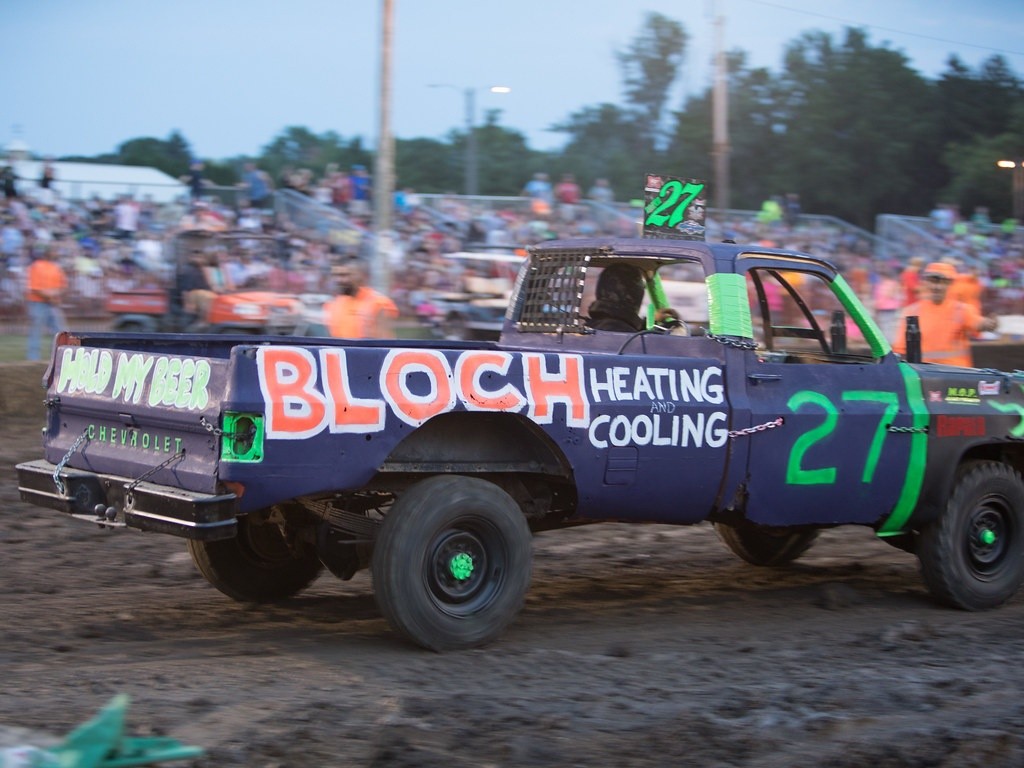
xmin=923 ymin=263 xmax=957 ymax=280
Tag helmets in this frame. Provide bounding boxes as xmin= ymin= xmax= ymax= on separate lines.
xmin=596 ymin=263 xmax=645 ymax=315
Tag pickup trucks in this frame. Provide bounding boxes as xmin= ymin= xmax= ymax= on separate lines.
xmin=16 ymin=234 xmax=1024 ymax=654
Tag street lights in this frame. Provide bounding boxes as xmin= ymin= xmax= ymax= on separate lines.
xmin=998 ymin=156 xmax=1023 ymax=222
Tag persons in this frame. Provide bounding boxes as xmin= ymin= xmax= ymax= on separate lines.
xmin=0 ymin=164 xmax=1024 ymax=348
xmin=891 ymin=263 xmax=996 ymax=369
xmin=25 ymin=244 xmax=71 ymax=362
